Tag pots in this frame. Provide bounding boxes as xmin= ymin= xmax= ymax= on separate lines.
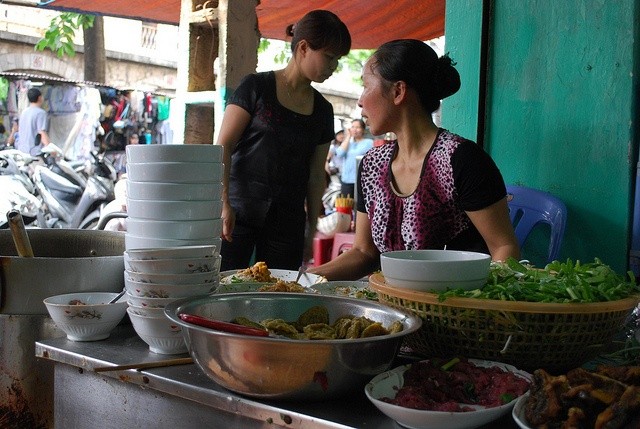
xmin=1 ymin=228 xmax=126 ymax=317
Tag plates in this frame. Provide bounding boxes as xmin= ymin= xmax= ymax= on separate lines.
xmin=512 ymin=390 xmax=536 ymax=429
xmin=220 ymin=268 xmax=330 ymax=286
xmin=363 ymin=358 xmax=533 ymax=429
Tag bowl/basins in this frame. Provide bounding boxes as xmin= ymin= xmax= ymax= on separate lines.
xmin=123 ymin=219 xmax=223 ymax=240
xmin=380 ymin=248 xmax=492 ymax=291
xmin=124 ymin=200 xmax=222 ymax=218
xmin=43 ymin=290 xmax=125 ymax=343
xmin=126 ymin=162 xmax=223 ymax=185
xmin=164 ymin=290 xmax=423 ymax=402
xmin=123 ymin=142 xmax=225 ymax=163
xmin=122 ymin=251 xmax=219 ymax=313
xmin=127 ymin=308 xmax=189 ymax=357
xmin=125 ymin=181 xmax=226 ymax=202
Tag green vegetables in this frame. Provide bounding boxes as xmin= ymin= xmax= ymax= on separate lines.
xmin=381 ymin=257 xmax=637 ymax=357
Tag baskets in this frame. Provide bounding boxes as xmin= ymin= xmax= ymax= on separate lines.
xmin=368 ymin=266 xmax=639 ymax=362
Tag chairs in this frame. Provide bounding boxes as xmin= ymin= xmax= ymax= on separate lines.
xmin=459 ymin=182 xmax=568 ymax=269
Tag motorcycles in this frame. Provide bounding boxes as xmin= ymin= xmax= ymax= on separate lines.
xmin=0 ymin=125 xmax=117 ymax=230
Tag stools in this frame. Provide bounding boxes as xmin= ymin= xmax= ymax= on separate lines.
xmin=330 ymin=232 xmax=356 ymax=261
xmin=304 ymin=231 xmax=333 ymax=267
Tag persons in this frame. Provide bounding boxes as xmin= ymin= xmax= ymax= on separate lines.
xmin=20 ymin=89 xmax=49 ymax=151
xmin=216 ymin=10 xmax=350 ymax=271
xmin=337 ymin=119 xmax=373 ymax=199
xmin=127 ymin=132 xmax=139 ymax=146
xmin=101 ymin=121 xmax=124 ymax=151
xmin=304 ymin=38 xmax=517 ymax=280
xmin=325 ymin=127 xmax=345 ymax=189
xmin=7 ymin=119 xmax=19 ymax=149
